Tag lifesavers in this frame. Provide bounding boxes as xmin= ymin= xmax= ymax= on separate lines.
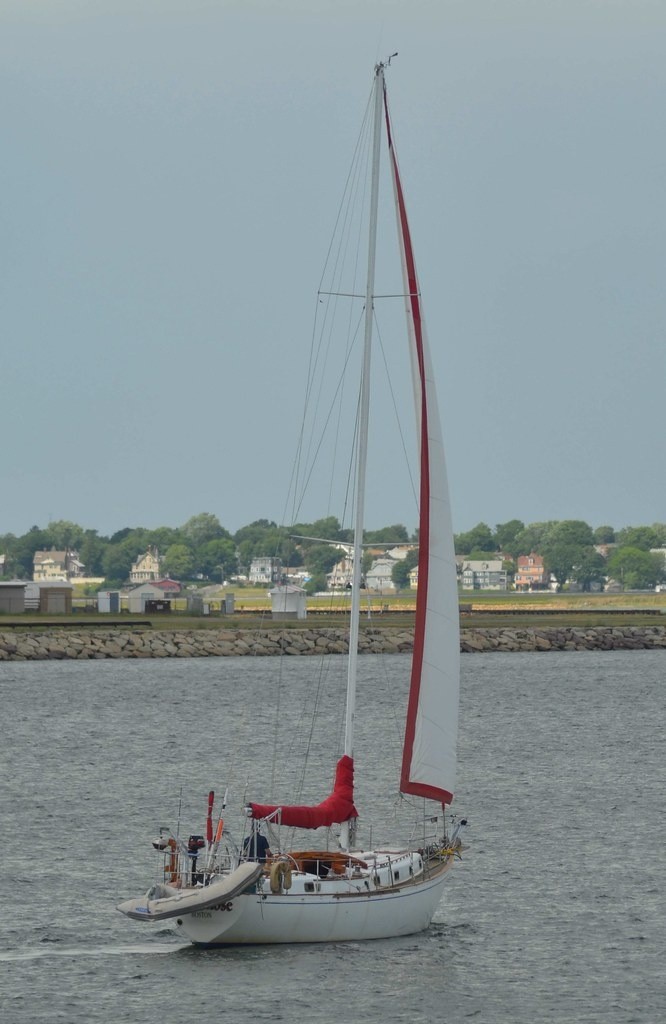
xmin=269 ymin=861 xmax=294 ymax=890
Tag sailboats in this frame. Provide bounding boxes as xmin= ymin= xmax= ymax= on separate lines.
xmin=115 ymin=51 xmax=469 ymax=949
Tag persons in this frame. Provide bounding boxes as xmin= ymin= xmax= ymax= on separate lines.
xmin=243 ymin=822 xmax=273 ymax=863
